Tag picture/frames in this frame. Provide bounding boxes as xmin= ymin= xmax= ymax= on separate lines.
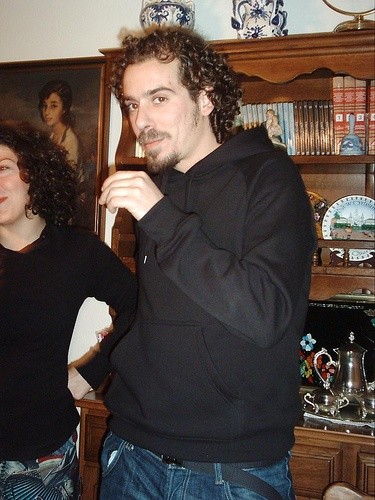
xmin=1 ymin=56 xmax=112 ymax=243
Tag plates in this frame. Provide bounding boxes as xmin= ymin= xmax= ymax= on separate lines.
xmin=322 ymin=195 xmax=375 ymax=262
xmin=306 ymin=189 xmax=330 ymax=241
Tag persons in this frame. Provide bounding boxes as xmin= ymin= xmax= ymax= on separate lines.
xmin=264 ymin=108 xmax=287 ymax=149
xmin=0 ymin=118 xmax=139 ymax=500
xmin=91 ymin=26 xmax=318 ymax=500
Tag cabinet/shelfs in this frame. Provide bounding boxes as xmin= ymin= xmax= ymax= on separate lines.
xmin=71 ymin=29 xmax=374 ymax=500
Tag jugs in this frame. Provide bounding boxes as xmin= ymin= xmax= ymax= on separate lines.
xmin=302 ymin=329 xmax=375 ymax=433
